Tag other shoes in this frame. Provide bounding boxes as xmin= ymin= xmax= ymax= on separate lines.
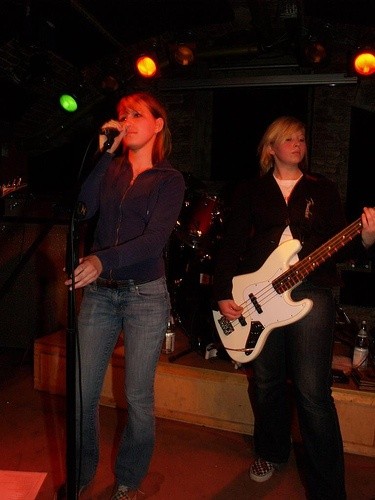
xmin=249 ymin=458 xmax=279 ymax=482
xmin=53 ymin=478 xmax=88 ymax=500
xmin=109 ymin=482 xmax=145 ymax=500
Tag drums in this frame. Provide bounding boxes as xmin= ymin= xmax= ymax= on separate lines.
xmin=173 ymin=189 xmax=228 ymax=250
xmin=172 ymin=257 xmax=212 ymax=355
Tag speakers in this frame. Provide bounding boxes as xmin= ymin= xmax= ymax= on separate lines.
xmin=0 ymin=215 xmax=99 ymax=356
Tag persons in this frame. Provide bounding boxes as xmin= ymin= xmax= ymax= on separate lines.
xmin=211 ymin=115 xmax=375 ymax=500
xmin=58 ymin=93 xmax=184 ymax=500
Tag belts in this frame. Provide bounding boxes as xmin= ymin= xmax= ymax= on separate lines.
xmin=89 ymin=275 xmax=153 ymax=289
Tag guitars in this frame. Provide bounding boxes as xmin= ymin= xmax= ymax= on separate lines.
xmin=207 ymin=206 xmax=375 ymax=368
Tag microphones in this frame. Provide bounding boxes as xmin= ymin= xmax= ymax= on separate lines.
xmin=95 ymin=127 xmax=120 ymax=138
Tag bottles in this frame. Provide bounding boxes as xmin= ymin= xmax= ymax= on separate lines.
xmin=161 ymin=320 xmax=175 ymax=353
xmin=352 ymin=320 xmax=368 ymax=370
